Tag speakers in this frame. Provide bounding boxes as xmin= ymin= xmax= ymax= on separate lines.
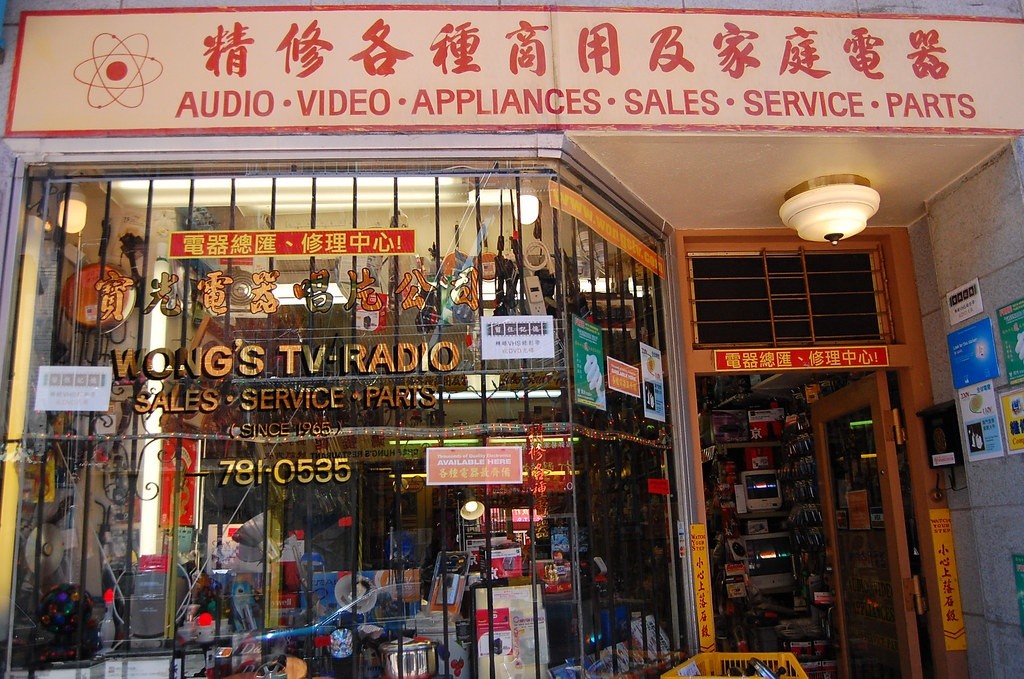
xmin=734 ymin=485 xmax=746 ymax=514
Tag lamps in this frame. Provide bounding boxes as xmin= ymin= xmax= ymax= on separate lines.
xmin=779 ymin=174 xmax=880 ymax=247
xmin=57 ymin=169 xmax=87 ymax=234
xmin=456 ymin=487 xmax=486 ymax=550
xmin=513 ymin=172 xmax=540 ymax=225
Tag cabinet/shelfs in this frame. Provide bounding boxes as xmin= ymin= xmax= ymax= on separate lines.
xmin=713 ymin=441 xmax=809 ymax=610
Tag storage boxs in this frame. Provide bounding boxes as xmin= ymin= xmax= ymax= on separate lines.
xmin=660 ymin=652 xmax=808 ymax=679
xmin=778 ymin=637 xmax=837 ymax=679
xmin=697 ymin=407 xmax=785 ymax=468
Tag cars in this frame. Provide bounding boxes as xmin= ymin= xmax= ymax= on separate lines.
xmin=230 ymin=574 xmax=603 ymax=679
xmin=10 ymin=598 xmax=55 ymax=645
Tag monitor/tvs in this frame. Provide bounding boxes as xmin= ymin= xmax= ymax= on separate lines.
xmin=741 ymin=470 xmax=782 ymax=513
